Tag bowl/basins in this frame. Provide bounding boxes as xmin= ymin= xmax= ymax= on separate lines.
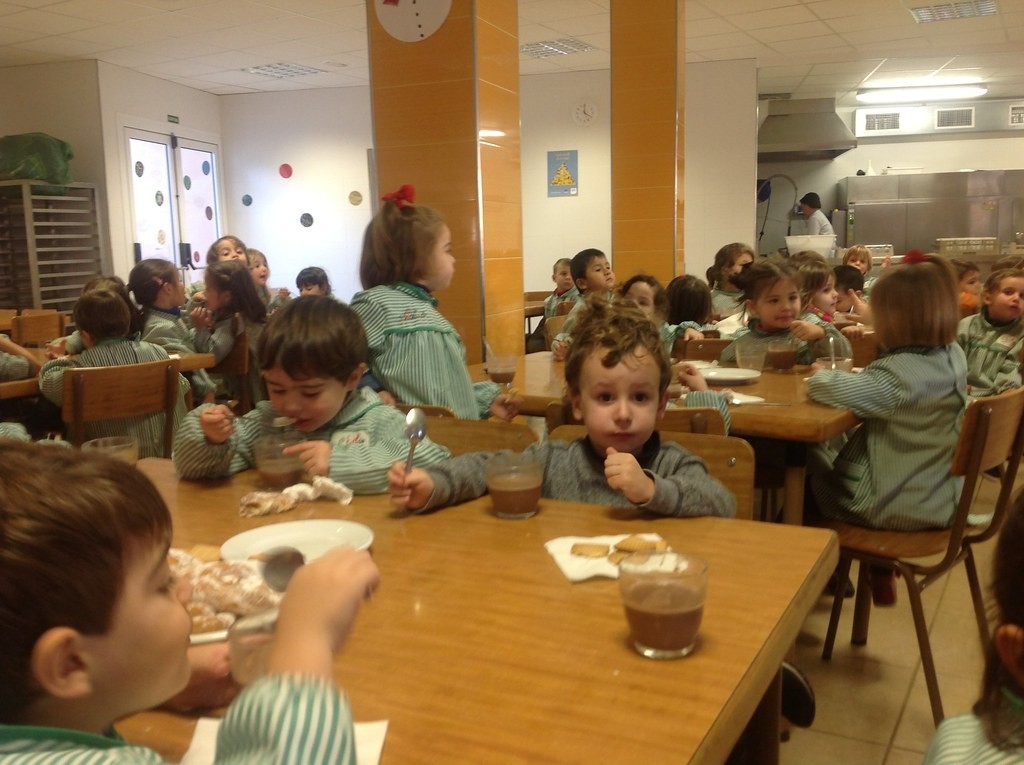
xmin=668 ymin=384 xmax=690 ymax=397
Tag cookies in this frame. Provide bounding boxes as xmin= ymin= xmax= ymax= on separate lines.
xmin=570 ymin=536 xmax=669 ymax=568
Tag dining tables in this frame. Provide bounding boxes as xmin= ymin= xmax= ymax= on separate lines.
xmin=524 ymin=301 xmax=544 ymax=317
xmin=129 ymin=456 xmax=841 ymax=764
xmin=469 ymin=348 xmax=861 ymax=529
xmin=0 ymin=348 xmax=214 ymax=402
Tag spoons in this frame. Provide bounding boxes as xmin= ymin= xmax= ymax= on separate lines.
xmin=254 ymin=433 xmax=305 ymax=487
xmin=400 ymin=407 xmax=427 ymax=512
xmin=264 ymin=550 xmax=303 ymax=592
xmin=207 ymin=411 xmax=296 ymax=426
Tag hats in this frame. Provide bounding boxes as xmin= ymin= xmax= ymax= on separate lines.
xmin=800 ymin=192 xmax=822 ymax=209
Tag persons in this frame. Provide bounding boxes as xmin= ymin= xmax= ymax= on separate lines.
xmin=801 ymin=192 xmax=837 ymax=241
xmin=349 ymin=186 xmax=517 ymax=422
xmin=0 ymin=236 xmax=1024 ymax=765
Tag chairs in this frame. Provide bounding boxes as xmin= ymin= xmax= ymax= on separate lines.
xmin=0 ymin=302 xmax=1023 ymax=728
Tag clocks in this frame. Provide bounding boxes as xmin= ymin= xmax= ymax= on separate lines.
xmin=572 ymin=101 xmax=597 ymax=125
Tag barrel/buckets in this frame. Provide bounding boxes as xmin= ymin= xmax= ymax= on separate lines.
xmin=783 ymin=235 xmax=837 ymax=269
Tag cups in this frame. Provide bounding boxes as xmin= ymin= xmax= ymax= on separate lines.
xmin=768 ymin=342 xmax=798 ymax=373
xmin=486 ymin=354 xmax=517 ymax=386
xmin=834 ymin=313 xmax=857 ymax=331
xmin=81 ymin=435 xmax=138 ymax=472
xmin=484 ymin=456 xmax=543 ymax=520
xmin=619 ymin=548 xmax=706 ymax=659
xmin=228 ymin=609 xmax=276 ymax=685
xmin=816 ymin=357 xmax=853 ymax=375
xmin=735 ymin=343 xmax=768 ymax=384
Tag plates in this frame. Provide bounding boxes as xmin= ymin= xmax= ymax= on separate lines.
xmin=187 ymin=608 xmax=279 ymax=644
xmin=220 ymin=519 xmax=375 ymax=563
xmin=698 ymin=367 xmax=761 ymax=385
xmin=679 ymin=393 xmax=763 ymax=404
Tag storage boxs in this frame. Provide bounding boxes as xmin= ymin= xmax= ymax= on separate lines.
xmin=784 ymin=233 xmax=838 ymax=258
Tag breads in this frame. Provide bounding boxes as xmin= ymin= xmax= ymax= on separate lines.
xmin=165 ymin=553 xmax=275 ymax=636
